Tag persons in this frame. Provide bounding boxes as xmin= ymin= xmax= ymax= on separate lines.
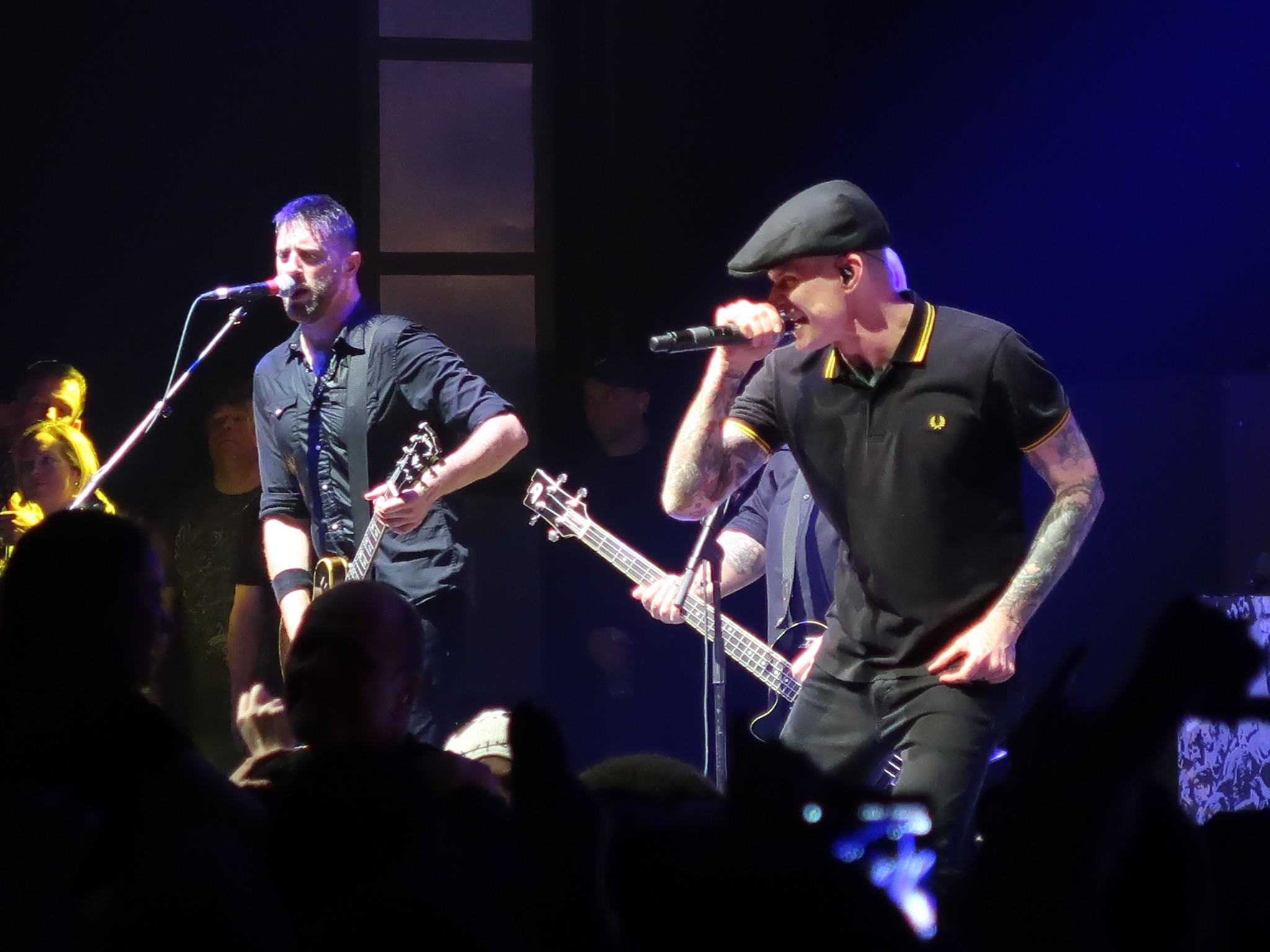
xmin=628 ymin=246 xmax=910 ymax=728
xmin=252 ymin=196 xmax=529 ymax=747
xmin=0 ymin=507 xmax=512 ymax=952
xmin=659 ymin=179 xmax=1107 ymax=838
xmin=537 ymin=341 xmax=700 ymax=756
xmin=508 ymin=591 xmax=1270 ymax=952
xmin=0 ymin=359 xmax=90 ymax=429
xmin=235 ymin=583 xmax=553 ymax=951
xmin=152 ymin=383 xmax=256 ymax=779
xmin=0 ymin=419 xmax=118 ymax=572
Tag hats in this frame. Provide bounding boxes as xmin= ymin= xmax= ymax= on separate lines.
xmin=726 ymin=179 xmax=893 ymax=280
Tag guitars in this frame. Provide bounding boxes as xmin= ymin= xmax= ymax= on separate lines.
xmin=279 ymin=421 xmax=446 ymax=682
xmin=521 ymin=465 xmax=829 ymax=744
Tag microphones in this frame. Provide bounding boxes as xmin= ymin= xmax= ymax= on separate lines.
xmin=644 ymin=315 xmax=796 ymax=358
xmin=201 ymin=274 xmax=297 ymax=304
